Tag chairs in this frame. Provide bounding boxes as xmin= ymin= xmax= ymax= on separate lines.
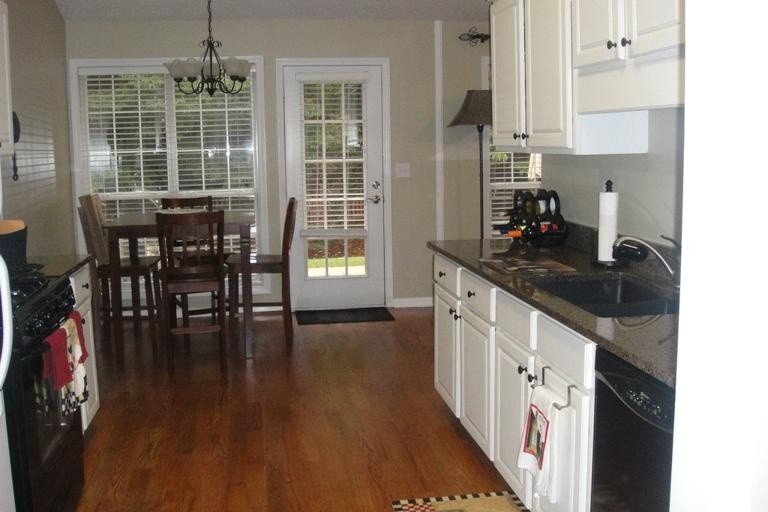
xmin=77 ymin=193 xmax=297 ymax=374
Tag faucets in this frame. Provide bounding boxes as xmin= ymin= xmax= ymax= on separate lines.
xmin=614 ymin=234 xmax=681 ymax=289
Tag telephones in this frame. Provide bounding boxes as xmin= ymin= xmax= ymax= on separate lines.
xmin=12 ymin=110 xmax=21 ymax=143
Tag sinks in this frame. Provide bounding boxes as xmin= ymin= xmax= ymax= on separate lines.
xmin=513 ymin=268 xmax=679 ymax=317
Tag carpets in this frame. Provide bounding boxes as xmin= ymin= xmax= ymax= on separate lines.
xmin=295 ymin=307 xmax=397 ymax=325
xmin=391 ymin=490 xmax=530 ymax=512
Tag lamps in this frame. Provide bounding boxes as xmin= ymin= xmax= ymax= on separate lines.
xmin=446 ymin=89 xmax=492 ymax=238
xmin=163 ymin=0 xmax=257 ymax=98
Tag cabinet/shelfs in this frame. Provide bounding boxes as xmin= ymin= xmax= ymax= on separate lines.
xmin=490 ymin=0 xmax=684 ymax=155
xmin=0 ymin=1 xmax=15 ymax=155
xmin=69 ymin=262 xmax=101 ymax=437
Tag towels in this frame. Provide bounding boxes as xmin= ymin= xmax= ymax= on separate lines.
xmin=44 ymin=313 xmax=89 ymax=413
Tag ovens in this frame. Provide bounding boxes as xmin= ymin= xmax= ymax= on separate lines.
xmin=13 ymin=325 xmax=89 ymax=467
